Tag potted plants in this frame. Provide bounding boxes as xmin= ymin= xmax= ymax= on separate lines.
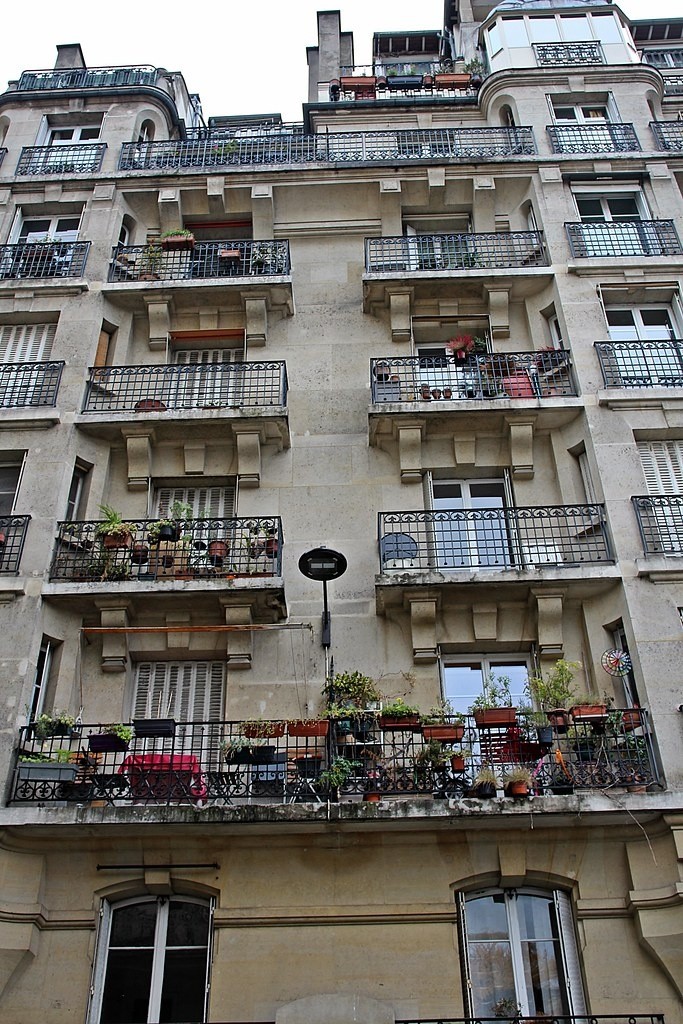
xmin=370 ymin=242 xmax=484 ymax=269
xmin=19 ymin=657 xmax=648 ymax=807
xmin=202 ymin=401 xmax=223 ymax=409
xmin=154 ymin=140 xmax=286 ymax=162
xmin=491 ymin=996 xmax=555 ymax=1024
xmin=230 ymin=405 xmax=240 ymax=408
xmin=329 ymin=53 xmax=485 ymax=100
xmin=59 ymin=500 xmax=280 ymax=581
xmin=122 ymin=157 xmax=141 ymax=168
xmin=23 ymin=235 xmax=62 ymax=276
xmin=119 ymin=226 xmax=284 ymax=282
xmin=63 ymin=163 xmax=74 ymax=171
xmin=133 ymin=384 xmax=167 ymax=412
xmin=0 ymin=528 xmax=5 ymax=543
xmin=373 ymin=334 xmax=565 ymax=400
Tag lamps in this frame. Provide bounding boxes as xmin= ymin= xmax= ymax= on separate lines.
xmin=298 ymin=547 xmax=346 ymax=647
